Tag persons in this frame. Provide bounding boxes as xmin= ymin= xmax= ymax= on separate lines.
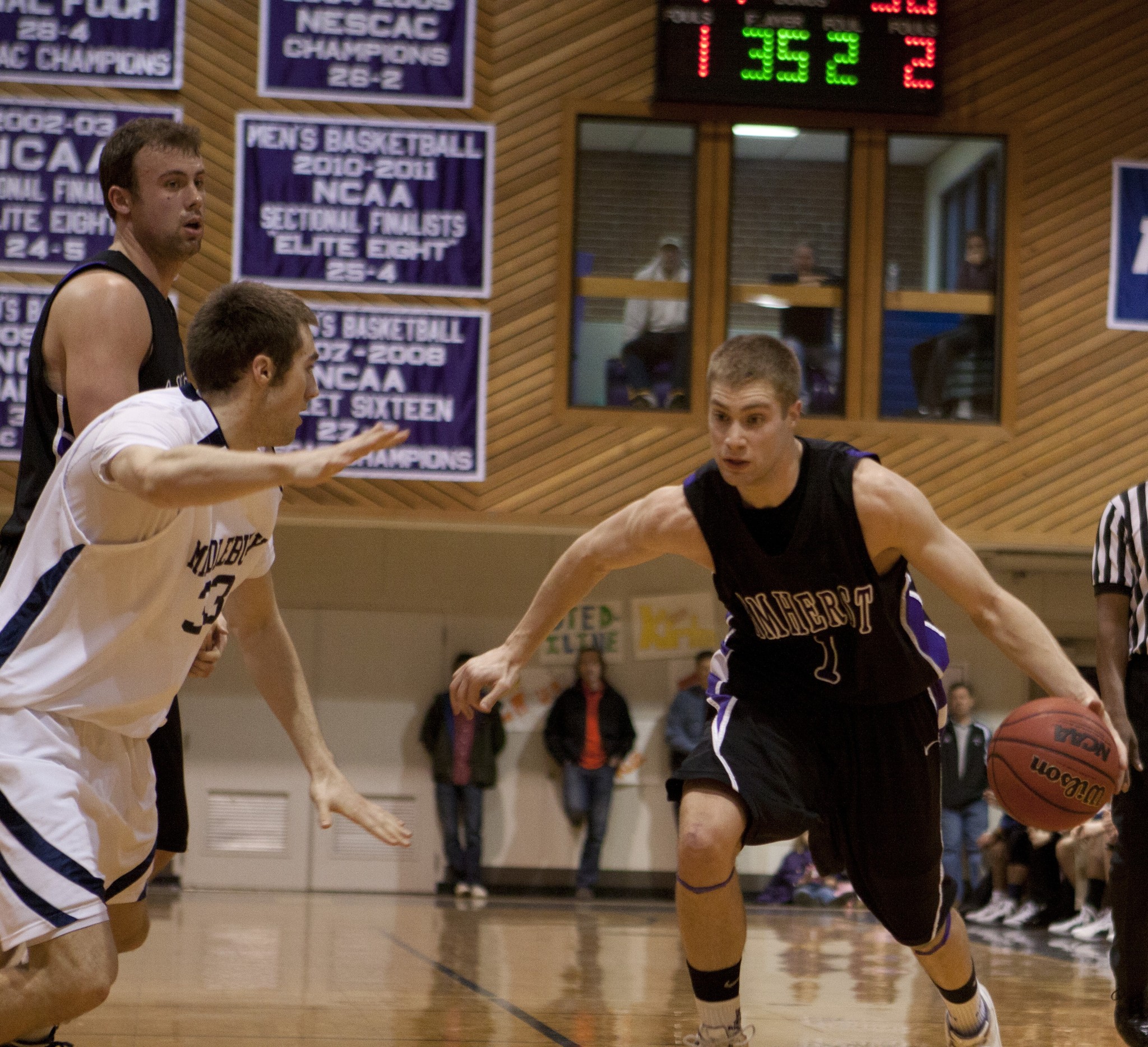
xmin=622 ymin=238 xmax=692 ymax=408
xmin=447 ymin=333 xmax=1129 ymax=1047
xmin=912 ymin=234 xmax=998 ymax=419
xmin=664 ymin=649 xmax=715 ymax=836
xmin=0 ymin=115 xmax=228 ymax=1039
xmin=756 ymin=678 xmax=1117 ymax=941
xmin=542 ymin=643 xmax=637 ymax=901
xmin=0 ymin=281 xmax=412 ymax=1047
xmin=766 ymin=239 xmax=847 ymax=412
xmin=417 ymin=650 xmax=507 ymax=900
xmin=1092 ymin=474 xmax=1148 ymax=1047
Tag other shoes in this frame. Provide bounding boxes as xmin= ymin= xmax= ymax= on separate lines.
xmin=577 ymin=886 xmax=593 ymax=900
xmin=470 ymin=885 xmax=487 ymax=897
xmin=454 ymin=882 xmax=469 ymax=895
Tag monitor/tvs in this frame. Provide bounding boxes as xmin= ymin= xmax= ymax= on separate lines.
xmin=654 ymin=0 xmax=942 ymax=114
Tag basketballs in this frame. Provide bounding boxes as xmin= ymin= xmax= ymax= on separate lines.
xmin=987 ymin=695 xmax=1121 ymax=833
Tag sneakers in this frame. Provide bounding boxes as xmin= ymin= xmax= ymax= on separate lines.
xmin=1071 ymin=911 xmax=1116 ymax=941
xmin=1048 ymin=907 xmax=1099 ymax=937
xmin=944 ymin=983 xmax=1002 ymax=1047
xmin=683 ymin=1024 xmax=756 ymax=1047
xmin=966 ymin=898 xmax=1011 ymax=922
xmin=979 ymin=902 xmax=1020 ymax=926
xmin=1003 ymin=903 xmax=1052 ymax=929
xmin=964 ymin=926 xmax=1110 ymax=963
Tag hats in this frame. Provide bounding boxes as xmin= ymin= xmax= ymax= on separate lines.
xmin=659 ymin=236 xmax=678 ymax=247
